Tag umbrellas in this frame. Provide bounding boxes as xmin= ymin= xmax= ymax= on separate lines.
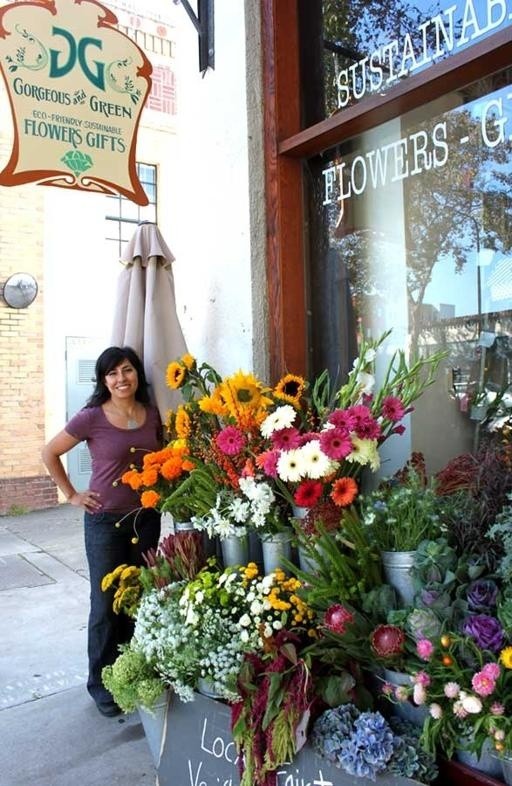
xmin=106 ymin=219 xmax=197 ymax=428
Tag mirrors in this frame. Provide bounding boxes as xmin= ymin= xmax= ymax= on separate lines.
xmin=3 ymin=272 xmax=38 ymax=309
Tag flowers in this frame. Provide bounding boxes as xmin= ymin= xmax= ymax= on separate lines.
xmin=98 ymin=328 xmax=511 ymax=785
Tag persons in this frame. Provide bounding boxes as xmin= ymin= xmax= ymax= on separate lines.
xmin=42 ymin=345 xmax=165 ymax=719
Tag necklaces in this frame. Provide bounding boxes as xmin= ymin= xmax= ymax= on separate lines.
xmin=109 ymin=400 xmax=143 ymax=429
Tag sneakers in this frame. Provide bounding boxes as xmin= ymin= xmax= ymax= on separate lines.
xmin=94 ymin=702 xmax=121 ymax=717
